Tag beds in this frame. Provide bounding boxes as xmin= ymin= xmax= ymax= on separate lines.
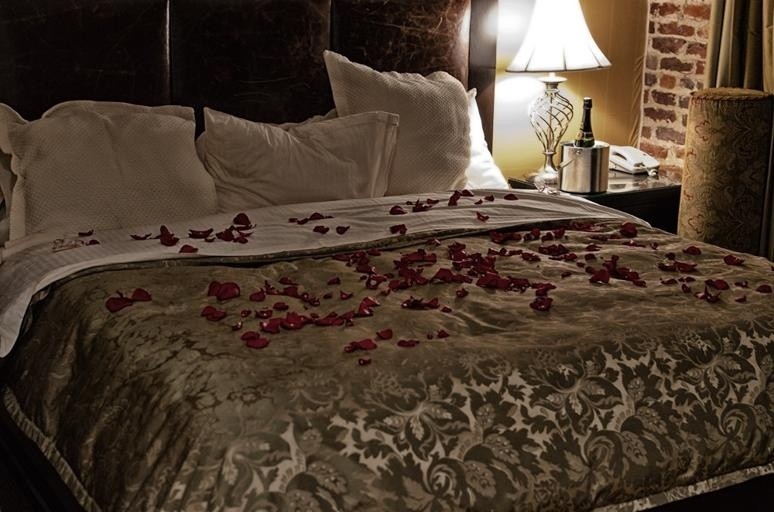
xmin=0 ymin=0 xmax=774 ymax=510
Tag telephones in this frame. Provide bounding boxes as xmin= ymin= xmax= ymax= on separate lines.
xmin=609 ymin=145 xmax=660 ymax=177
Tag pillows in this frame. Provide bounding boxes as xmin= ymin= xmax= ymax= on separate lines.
xmin=196 ymin=108 xmax=400 ymax=212
xmin=323 ymin=49 xmax=470 ymax=196
xmin=467 ymin=87 xmax=512 ymax=191
xmin=1 ymin=101 xmax=218 ymax=259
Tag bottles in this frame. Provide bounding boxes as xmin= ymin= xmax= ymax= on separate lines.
xmin=572 ymin=97 xmax=595 ymax=147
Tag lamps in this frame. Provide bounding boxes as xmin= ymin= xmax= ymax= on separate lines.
xmin=504 ymin=0 xmax=611 ymax=187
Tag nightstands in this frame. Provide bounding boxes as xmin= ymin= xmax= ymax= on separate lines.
xmin=510 ymin=165 xmax=681 ymax=228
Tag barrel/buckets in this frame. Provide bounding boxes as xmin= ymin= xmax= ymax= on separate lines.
xmin=555 ymin=138 xmax=610 ymax=194
